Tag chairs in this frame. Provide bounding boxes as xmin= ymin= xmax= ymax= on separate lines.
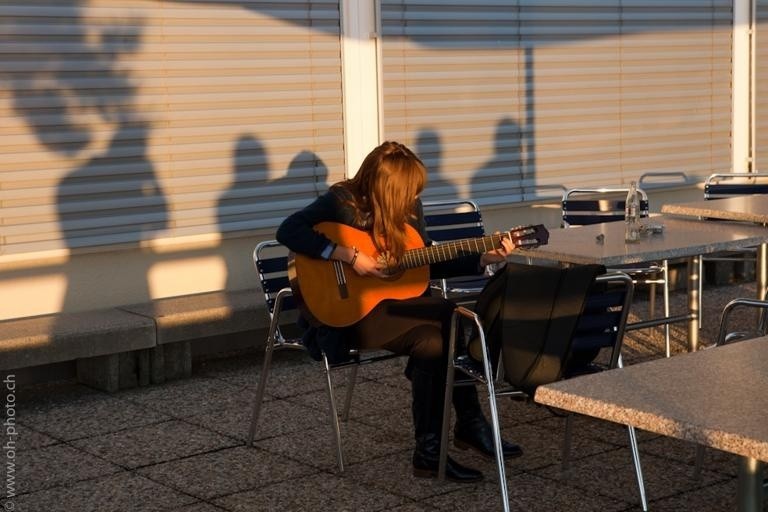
xmin=562 ymin=188 xmax=671 ymax=356
xmin=698 ymin=173 xmax=768 ymax=328
xmin=420 ymin=198 xmax=498 ymax=299
xmin=438 ymin=261 xmax=649 ymax=511
xmin=717 ymin=299 xmax=768 ymax=346
xmin=248 ymin=241 xmax=412 ymax=478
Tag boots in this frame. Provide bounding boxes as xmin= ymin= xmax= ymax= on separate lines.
xmin=447 ymin=336 xmax=522 ymax=458
xmin=412 ymin=367 xmax=484 ymax=482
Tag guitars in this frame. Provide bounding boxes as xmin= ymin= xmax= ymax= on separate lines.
xmin=287 ymin=221 xmax=549 ymax=332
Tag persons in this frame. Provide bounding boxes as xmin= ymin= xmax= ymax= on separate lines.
xmin=275 ymin=140 xmax=524 ymax=483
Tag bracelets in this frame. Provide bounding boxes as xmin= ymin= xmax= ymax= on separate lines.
xmin=350 ymin=247 xmax=359 ymax=267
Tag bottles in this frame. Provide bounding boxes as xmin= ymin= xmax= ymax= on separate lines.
xmin=623 ymin=180 xmax=649 ymax=245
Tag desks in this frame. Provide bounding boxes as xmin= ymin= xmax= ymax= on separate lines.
xmin=659 ymin=193 xmax=766 ymax=334
xmin=485 ymin=211 xmax=767 ymax=352
xmin=534 ymin=334 xmax=767 ymax=511
